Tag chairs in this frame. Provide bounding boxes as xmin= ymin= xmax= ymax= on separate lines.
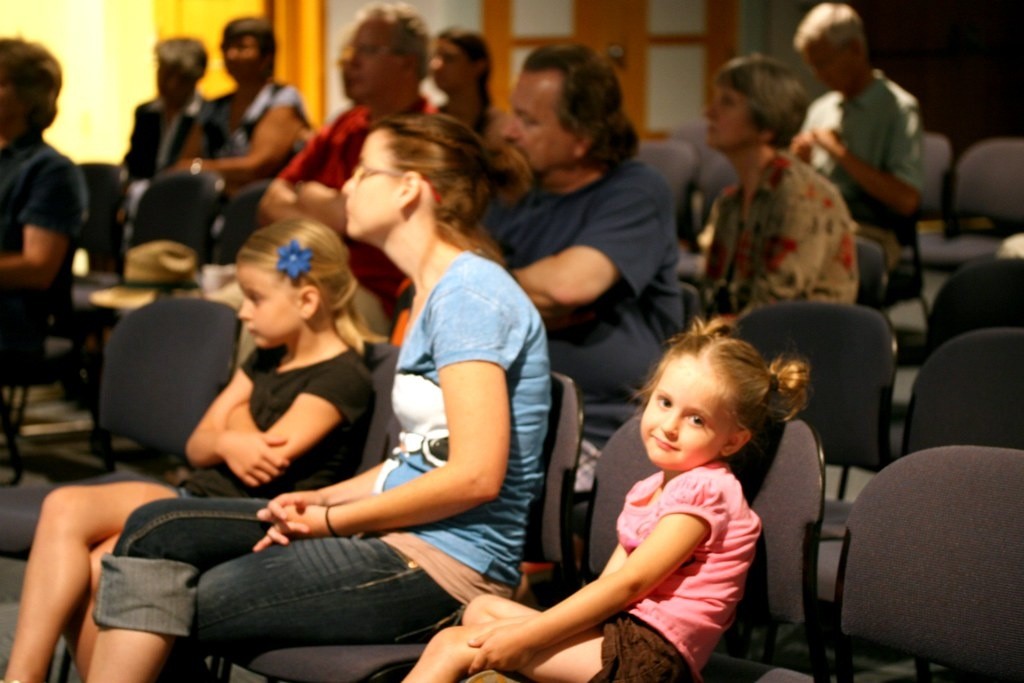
xmin=834 ymin=446 xmax=1024 ymax=683
xmin=732 ymin=302 xmax=898 ymax=648
xmin=128 ymin=168 xmax=229 ymax=275
xmin=211 ymin=374 xmax=582 ymax=683
xmin=218 ymin=183 xmax=271 ymax=268
xmin=899 ymin=328 xmax=1024 ymax=457
xmin=901 ymin=257 xmax=1024 ymax=455
xmin=917 ymin=132 xmax=951 ymax=219
xmin=672 ymin=118 xmax=741 ymax=236
xmin=953 ymin=139 xmax=1024 ymax=241
xmin=58 ymin=339 xmax=404 ymax=683
xmin=918 ymin=227 xmax=1002 ymax=273
xmin=834 ymin=237 xmax=900 ymax=499
xmin=0 ymin=298 xmax=242 ymax=683
xmin=640 ymin=138 xmax=705 ymax=274
xmin=581 ymin=418 xmax=827 ymax=683
xmin=0 ymin=164 xmax=125 ymax=478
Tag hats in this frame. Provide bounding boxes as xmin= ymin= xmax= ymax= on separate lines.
xmin=87 ymin=240 xmax=202 ymax=310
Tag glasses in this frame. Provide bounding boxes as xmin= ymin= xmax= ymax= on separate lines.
xmin=357 ymin=163 xmax=441 ymax=202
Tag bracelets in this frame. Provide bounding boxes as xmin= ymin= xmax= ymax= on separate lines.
xmin=325 ymin=506 xmax=341 ymax=539
xmin=189 ymin=158 xmax=204 ymax=176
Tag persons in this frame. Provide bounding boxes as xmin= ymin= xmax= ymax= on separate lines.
xmin=135 ymin=18 xmax=310 ymax=255
xmin=702 ymin=51 xmax=862 ymax=316
xmin=0 ymin=36 xmax=93 ymax=385
xmin=430 ymin=27 xmax=511 ymax=146
xmin=1 ymin=214 xmax=388 ymax=683
xmin=259 ymin=3 xmax=441 ymax=338
xmin=110 ymin=36 xmax=209 ymax=248
xmin=788 ymin=2 xmax=927 ymax=306
xmin=398 ymin=313 xmax=814 ymax=682
xmin=82 ymin=111 xmax=552 ymax=680
xmin=484 ymin=40 xmax=688 ymax=507
